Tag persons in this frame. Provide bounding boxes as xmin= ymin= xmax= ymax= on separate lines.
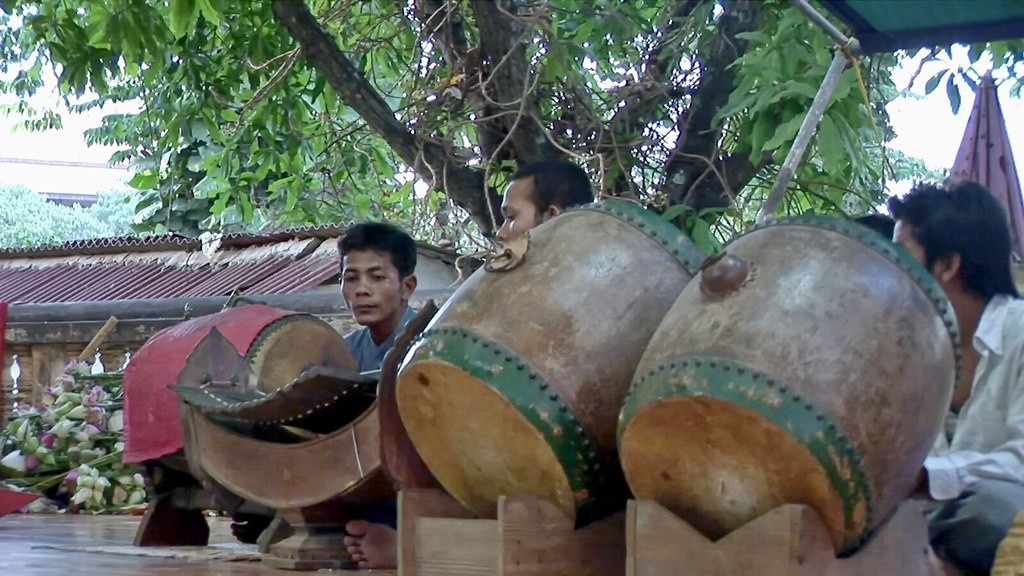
xmin=336 ymin=221 xmax=432 ymax=568
xmin=496 ymin=162 xmax=596 ymax=245
xmin=888 ymin=181 xmax=1024 ymax=571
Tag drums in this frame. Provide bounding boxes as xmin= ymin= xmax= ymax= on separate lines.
xmin=619 ymin=199 xmax=963 ymax=560
xmin=125 ymin=304 xmax=357 ymax=472
xmin=392 ymin=197 xmax=703 ymax=534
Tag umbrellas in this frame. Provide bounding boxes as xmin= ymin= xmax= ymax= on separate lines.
xmin=944 ymin=69 xmax=1024 ymax=264
